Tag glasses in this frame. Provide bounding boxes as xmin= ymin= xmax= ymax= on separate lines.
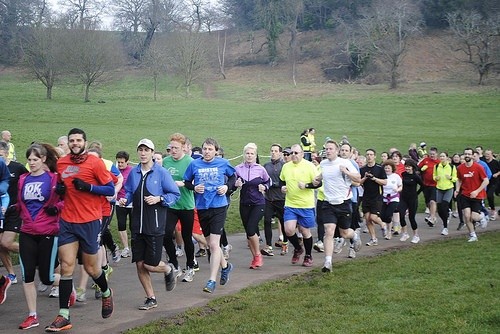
xmin=284 ymin=154 xmax=290 ymax=156
xmin=290 ymin=151 xmax=301 ymax=154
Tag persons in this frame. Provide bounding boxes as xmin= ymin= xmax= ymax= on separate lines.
xmin=454 ymin=148 xmax=489 ymax=242
xmin=418 ymin=142 xmax=500 ymax=231
xmin=313 ymin=140 xmax=362 ymax=273
xmin=45 ymin=128 xmax=114 ymax=332
xmin=162 ymin=133 xmax=198 ymax=282
xmin=6 ymin=142 xmax=76 ymax=330
xmin=152 ymin=145 xmax=232 ymax=260
xmin=120 ymin=138 xmax=176 ymax=310
xmin=182 ymin=138 xmax=237 ymax=293
xmin=234 ymin=143 xmax=272 ymax=269
xmin=76 ymin=140 xmax=132 ymax=302
xmin=260 ymin=143 xmax=425 ymax=258
xmin=0 ymin=131 xmax=71 ymax=305
xmin=300 ymin=127 xmax=316 ymax=160
xmin=433 ymin=152 xmax=458 ymax=235
xmin=279 ymin=143 xmax=323 ymax=267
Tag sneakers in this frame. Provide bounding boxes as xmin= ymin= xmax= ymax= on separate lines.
xmin=91 ymin=264 xmax=113 ymax=288
xmin=138 ymin=297 xmax=157 ymax=309
xmin=38 ymin=282 xmax=48 ymax=291
xmin=111 ymin=243 xmax=121 ymax=263
xmin=91 ymin=269 xmax=109 ymax=299
xmin=164 ymin=263 xmax=177 ymax=292
xmin=176 ymin=266 xmax=182 ymax=276
xmin=101 ymin=287 xmax=114 ymax=319
xmin=7 ymin=273 xmax=17 ymax=283
xmin=76 ymin=288 xmax=86 ymax=301
xmin=175 ymin=248 xmax=183 ymax=256
xmin=49 ymin=286 xmax=59 ymax=297
xmin=19 ymin=314 xmax=40 ymax=329
xmin=44 ymin=315 xmax=73 ymax=332
xmin=121 ymin=248 xmax=131 ymax=257
xmin=182 ymin=204 xmax=500 ymax=292
xmin=0 ymin=276 xmax=11 ymax=304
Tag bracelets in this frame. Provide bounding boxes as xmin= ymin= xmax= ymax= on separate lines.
xmin=456 ymin=190 xmax=459 ymax=193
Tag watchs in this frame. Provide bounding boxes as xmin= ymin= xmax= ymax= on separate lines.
xmin=160 ymin=196 xmax=164 ymax=202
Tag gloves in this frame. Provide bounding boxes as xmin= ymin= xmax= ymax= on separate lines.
xmin=71 ymin=177 xmax=92 ymax=192
xmin=5 ymin=206 xmax=16 ymax=217
xmin=44 ymin=205 xmax=58 ymax=216
xmin=55 ymin=182 xmax=66 ymax=194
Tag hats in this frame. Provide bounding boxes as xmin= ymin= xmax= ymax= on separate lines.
xmin=136 ymin=139 xmax=154 ymax=151
xmin=282 ymin=147 xmax=291 ymax=153
